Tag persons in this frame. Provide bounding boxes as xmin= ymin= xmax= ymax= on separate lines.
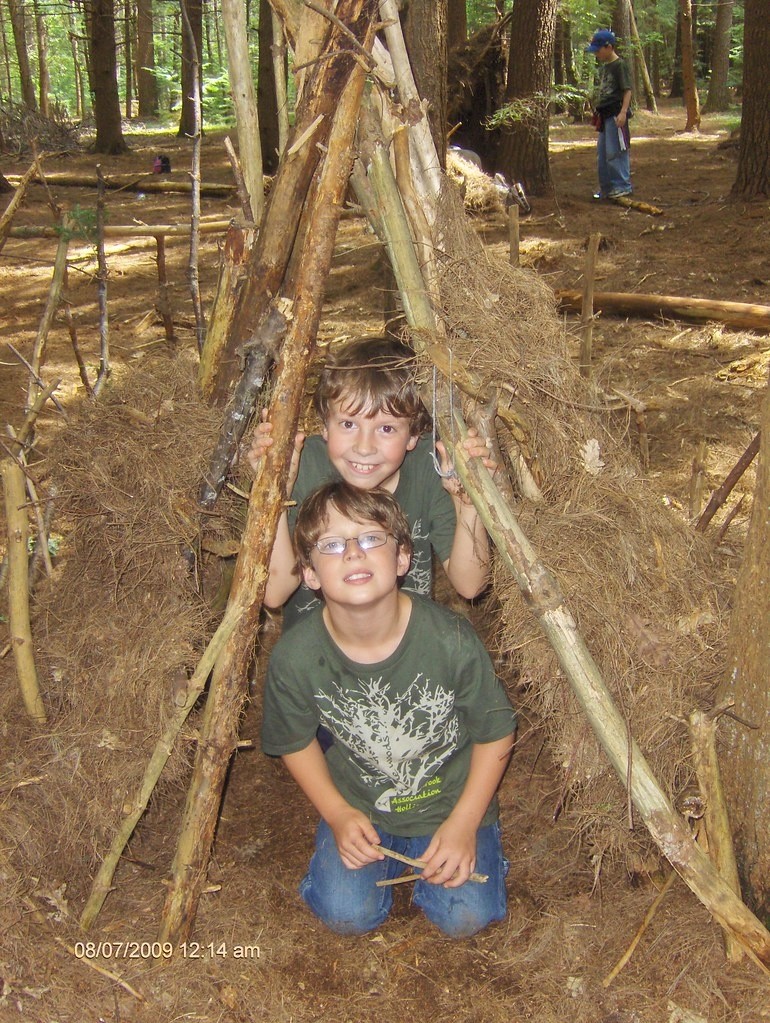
xmin=247 ymin=337 xmax=498 ymax=609
xmin=447 ymin=145 xmax=531 ymax=213
xmin=268 ymin=484 xmax=516 ymax=938
xmin=586 ymin=30 xmax=635 ymax=199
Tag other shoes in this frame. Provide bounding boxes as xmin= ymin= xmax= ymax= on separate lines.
xmin=606 ymin=189 xmax=630 ymax=199
xmin=593 ymin=188 xmax=611 ymax=199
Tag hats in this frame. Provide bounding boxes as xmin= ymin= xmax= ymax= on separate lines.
xmin=584 ymin=30 xmax=616 ymax=52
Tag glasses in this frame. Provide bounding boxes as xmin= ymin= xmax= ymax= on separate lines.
xmin=306 ymin=530 xmax=401 ymax=568
